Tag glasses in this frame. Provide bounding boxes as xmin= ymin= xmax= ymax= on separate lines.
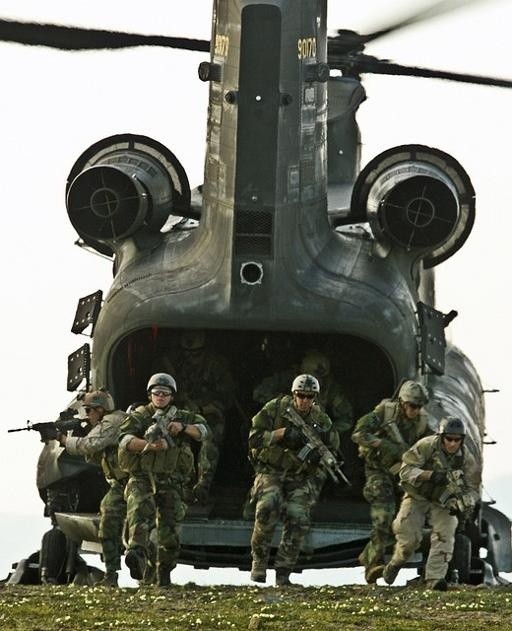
xmin=407 ymin=403 xmax=423 ymax=410
xmin=443 ymin=435 xmax=463 ymax=442
xmin=182 ymin=350 xmax=202 ymax=356
xmin=84 ymin=407 xmax=91 ymax=414
xmin=150 ymin=388 xmax=173 ymax=396
xmin=294 ymin=392 xmax=315 ymax=399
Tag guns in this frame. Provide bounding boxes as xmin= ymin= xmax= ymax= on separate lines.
xmin=382 ymin=420 xmax=406 ymax=444
xmin=9 ymin=418 xmax=89 ymax=442
xmin=434 ymin=449 xmax=474 ymax=521
xmin=283 ymin=408 xmax=350 ymax=488
xmin=141 ymin=410 xmax=175 ymax=456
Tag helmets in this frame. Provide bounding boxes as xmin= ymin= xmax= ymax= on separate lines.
xmin=399 ymin=380 xmax=428 ymax=404
xmin=292 ymin=374 xmax=321 ymax=395
xmin=181 ymin=330 xmax=207 ymax=350
xmin=82 ymin=391 xmax=114 ymax=412
xmin=439 ymin=416 xmax=464 ymax=436
xmin=146 ymin=373 xmax=177 ymax=393
xmin=300 ymin=351 xmax=330 ymax=376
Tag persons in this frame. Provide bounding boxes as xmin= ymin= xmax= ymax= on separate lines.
xmin=144 ymin=329 xmax=236 ymax=504
xmin=56 ymin=389 xmax=128 ymax=586
xmin=247 ymin=374 xmax=342 ymax=585
xmin=241 ymin=348 xmax=356 ymax=521
xmin=350 ymin=379 xmax=438 ymax=585
xmin=116 ymin=372 xmax=208 ymax=587
xmin=381 ymin=415 xmax=483 ymax=588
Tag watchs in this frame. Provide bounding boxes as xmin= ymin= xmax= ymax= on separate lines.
xmin=179 ymin=421 xmax=187 ymax=433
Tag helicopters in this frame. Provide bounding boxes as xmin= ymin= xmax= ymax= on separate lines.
xmin=0 ymin=0 xmax=512 ymax=587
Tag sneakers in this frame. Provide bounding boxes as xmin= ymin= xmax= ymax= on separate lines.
xmin=251 ymin=560 xmax=266 ymax=582
xmin=382 ymin=561 xmax=400 ymax=584
xmin=192 ymin=479 xmax=210 ymax=506
xmin=276 ymin=568 xmax=292 ymax=586
xmin=94 ymin=571 xmax=119 ymax=587
xmin=365 ymin=563 xmax=386 ymax=583
xmin=138 ymin=564 xmax=155 ymax=585
xmin=125 ymin=549 xmax=145 ymax=580
xmin=425 ymin=578 xmax=448 ymax=591
xmin=156 ymin=565 xmax=171 ymax=586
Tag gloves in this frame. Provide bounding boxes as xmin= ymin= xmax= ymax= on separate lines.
xmin=429 ymin=471 xmax=449 ymax=487
xmin=379 ymin=440 xmax=407 ymax=458
xmin=284 ymin=426 xmax=304 ymax=440
